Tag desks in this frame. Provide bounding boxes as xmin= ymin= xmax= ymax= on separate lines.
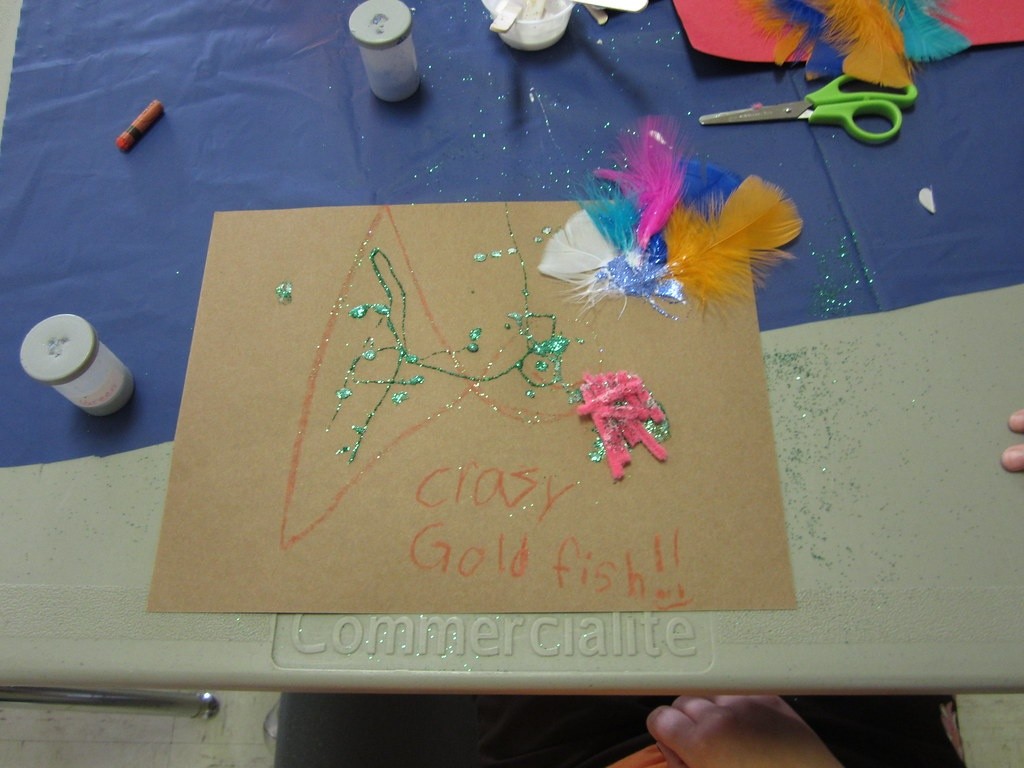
xmin=0 ymin=0 xmax=1024 ymax=721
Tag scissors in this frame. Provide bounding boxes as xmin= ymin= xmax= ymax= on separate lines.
xmin=697 ymin=66 xmax=920 ymax=147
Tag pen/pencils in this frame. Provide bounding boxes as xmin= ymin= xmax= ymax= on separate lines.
xmin=0 ymin=683 xmax=220 ymax=719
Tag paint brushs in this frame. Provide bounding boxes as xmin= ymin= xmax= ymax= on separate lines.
xmin=114 ymin=98 xmax=164 ymax=153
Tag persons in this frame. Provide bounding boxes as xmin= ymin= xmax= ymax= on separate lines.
xmin=1002 ymin=409 xmax=1024 ymax=471
xmin=274 ymin=694 xmax=967 ymax=768
